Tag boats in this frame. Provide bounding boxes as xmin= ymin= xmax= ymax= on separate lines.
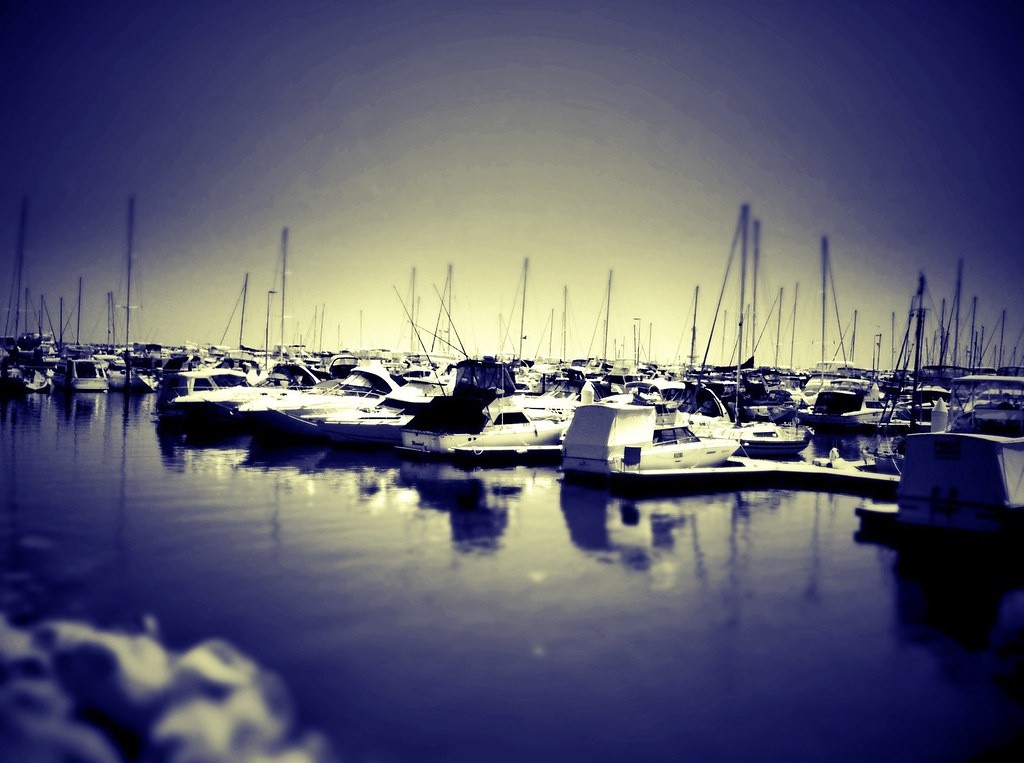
xmin=230 ymin=364 xmax=361 ymax=419
xmin=169 ymin=363 xmax=322 ymax=415
xmin=561 ymin=402 xmax=742 ymax=476
xmin=300 ymin=377 xmax=456 ymax=445
xmin=266 ymin=365 xmax=408 ymax=434
xmin=855 ymin=433 xmax=1024 ymax=539
xmin=400 ymin=405 xmax=572 ymax=455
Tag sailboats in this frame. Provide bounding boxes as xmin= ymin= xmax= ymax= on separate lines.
xmin=161 ymin=228 xmax=695 ymax=411
xmin=696 ymin=204 xmax=1024 ymax=454
xmin=0 ymin=194 xmax=159 ymax=393
